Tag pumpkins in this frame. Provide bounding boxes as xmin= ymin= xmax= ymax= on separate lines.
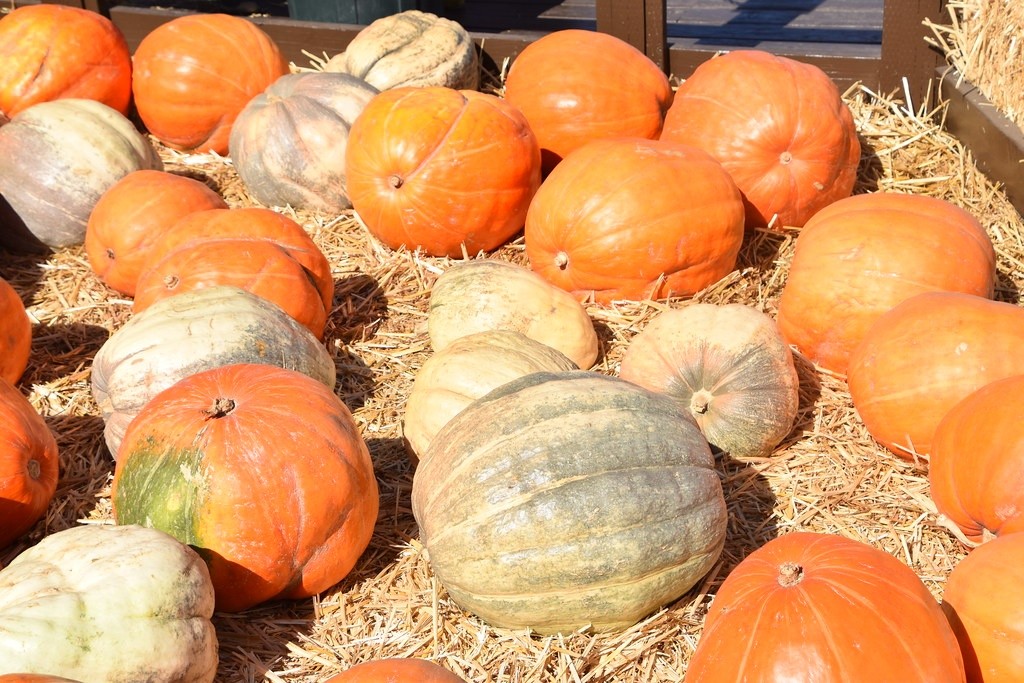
xmin=0 ymin=3 xmax=1024 ymax=683
